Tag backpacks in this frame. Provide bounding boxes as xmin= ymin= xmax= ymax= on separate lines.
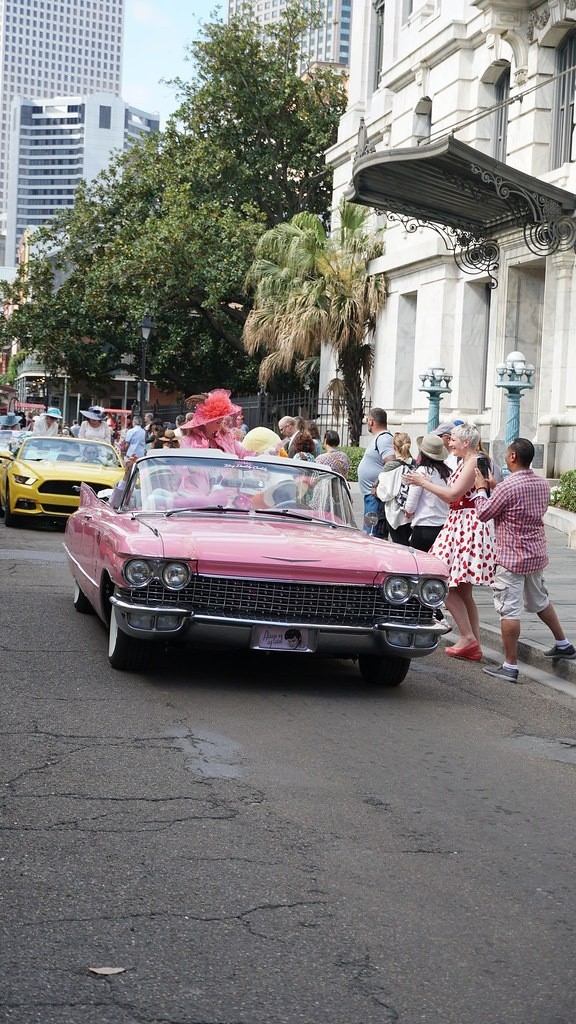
xmin=394 ymin=459 xmax=415 ymax=511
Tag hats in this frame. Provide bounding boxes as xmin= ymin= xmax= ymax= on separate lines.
xmin=42 ymin=408 xmax=63 ymax=419
xmin=429 ymin=423 xmax=456 ymax=436
xmin=179 ymin=387 xmax=242 ymax=430
xmin=453 ymin=420 xmax=464 ymax=427
xmin=0 ymin=412 xmax=22 ymax=427
xmin=150 ymin=418 xmax=180 ymax=441
xmin=79 ymin=406 xmax=108 ymax=421
xmin=416 ymin=434 xmax=449 ymax=460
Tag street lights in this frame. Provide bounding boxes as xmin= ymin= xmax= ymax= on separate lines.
xmin=134 ymin=310 xmax=158 ymax=430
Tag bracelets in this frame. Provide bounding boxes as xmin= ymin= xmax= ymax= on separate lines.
xmin=476 ymin=487 xmax=488 ymax=493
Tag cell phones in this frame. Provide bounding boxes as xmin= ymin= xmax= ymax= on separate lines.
xmin=477 ymin=457 xmax=489 ymax=480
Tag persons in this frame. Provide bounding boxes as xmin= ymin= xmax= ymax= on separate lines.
xmin=70 ymin=420 xmax=81 ymax=438
xmin=473 ymin=438 xmax=576 ymax=683
xmin=309 ymin=430 xmax=348 ymax=488
xmin=371 ymin=432 xmax=417 ymax=547
xmin=0 ymin=412 xmax=26 ymax=432
xmin=79 ymin=405 xmax=116 ymax=445
xmin=357 ymin=407 xmax=395 ymax=543
xmin=125 ymin=416 xmax=147 ymax=461
xmin=143 ymin=388 xmax=276 ymax=494
xmin=402 ymin=421 xmax=503 ymax=662
xmin=263 ymin=474 xmax=309 ymax=509
xmin=83 ymin=446 xmax=100 ymax=465
xmin=33 ymin=407 xmax=62 ymax=436
xmin=278 ymin=415 xmax=323 ymax=463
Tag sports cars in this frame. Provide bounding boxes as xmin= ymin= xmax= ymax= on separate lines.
xmin=0 ymin=435 xmax=141 ymax=528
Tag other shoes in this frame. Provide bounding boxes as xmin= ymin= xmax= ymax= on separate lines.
xmin=444 ymin=640 xmax=479 ymax=656
xmin=465 ymin=651 xmax=483 ymax=661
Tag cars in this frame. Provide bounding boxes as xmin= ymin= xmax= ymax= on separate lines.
xmin=60 ymin=447 xmax=453 ymax=688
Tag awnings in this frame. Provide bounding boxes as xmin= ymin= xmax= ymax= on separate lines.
xmin=342 ymin=135 xmax=576 ymax=275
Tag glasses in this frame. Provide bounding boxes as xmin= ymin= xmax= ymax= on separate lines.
xmin=48 ymin=415 xmax=59 ymax=420
xmin=280 ymin=423 xmax=290 ymax=433
xmin=85 ymin=450 xmax=98 ymax=454
xmin=437 ymin=433 xmax=451 ymax=438
xmin=394 ymin=432 xmax=400 ymax=444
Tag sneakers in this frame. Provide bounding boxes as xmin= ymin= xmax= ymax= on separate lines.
xmin=482 ymin=664 xmax=519 ymax=682
xmin=543 ymin=645 xmax=576 ymax=658
